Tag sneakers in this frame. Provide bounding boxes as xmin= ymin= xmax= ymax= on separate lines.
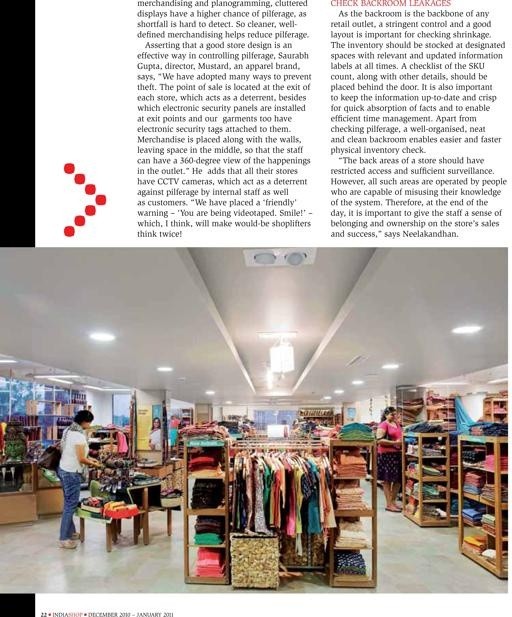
xmin=59 ymin=539 xmax=77 ymax=550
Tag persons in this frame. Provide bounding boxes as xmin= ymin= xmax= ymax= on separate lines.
xmin=376 ymin=406 xmax=404 ymax=512
xmin=169 ymin=413 xmax=181 ymax=450
xmin=148 ymin=416 xmax=161 ymax=450
xmin=57 ymin=409 xmax=103 ymax=548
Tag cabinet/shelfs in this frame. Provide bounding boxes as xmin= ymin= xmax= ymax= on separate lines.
xmin=294 ymin=407 xmax=342 ymax=434
xmin=183 ymin=428 xmax=378 ymax=590
xmin=369 ymin=393 xmax=508 ymax=579
xmin=0 ymin=390 xmax=192 ymax=551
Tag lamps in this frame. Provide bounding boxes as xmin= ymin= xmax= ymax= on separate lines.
xmin=266 ymin=332 xmax=296 ymax=378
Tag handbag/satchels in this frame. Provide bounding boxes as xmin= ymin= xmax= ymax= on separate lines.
xmin=37 ymin=446 xmax=60 ymax=470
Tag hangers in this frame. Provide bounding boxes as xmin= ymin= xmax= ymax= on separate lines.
xmin=231 ymin=438 xmax=326 ymax=460
